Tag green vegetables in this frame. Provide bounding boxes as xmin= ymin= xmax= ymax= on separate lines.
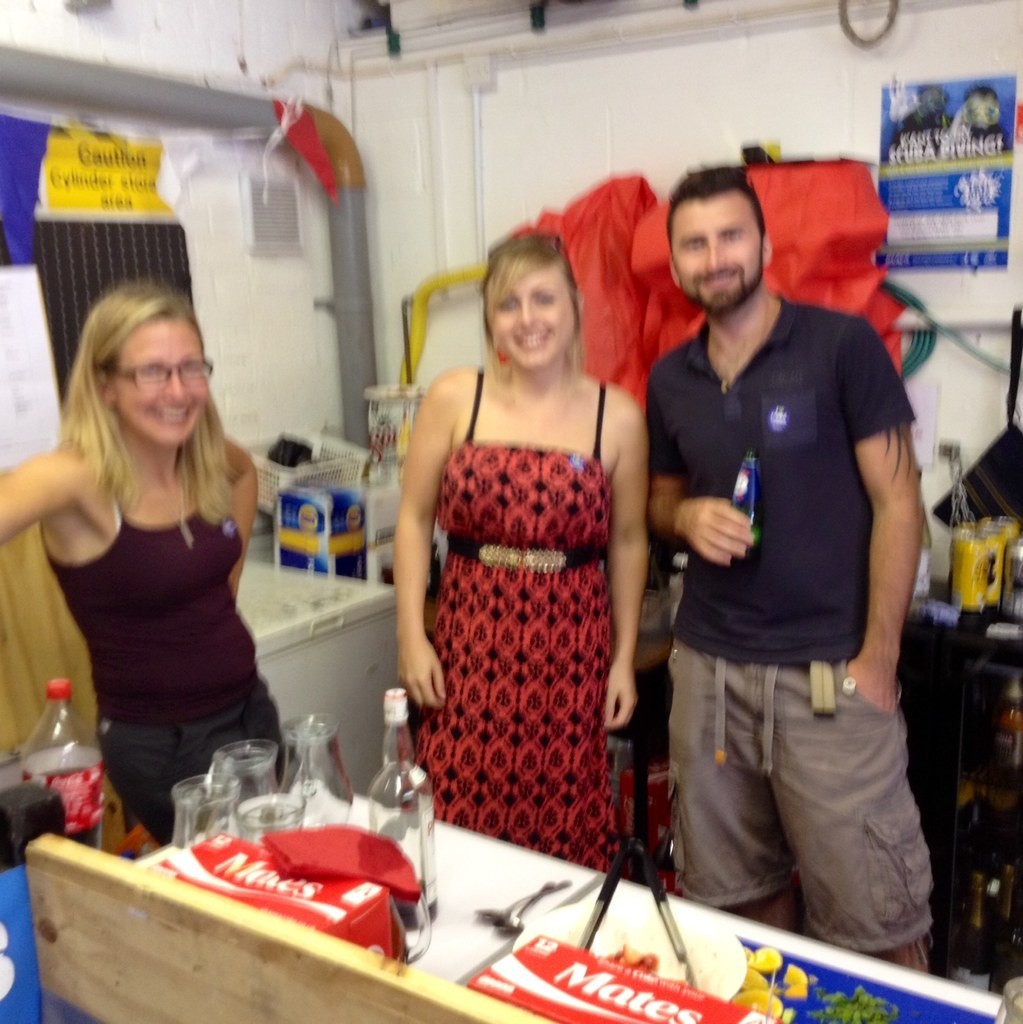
xmin=809 ymin=984 xmax=899 ymax=1024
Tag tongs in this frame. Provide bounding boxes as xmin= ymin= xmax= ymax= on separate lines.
xmin=578 ymin=833 xmax=698 ymax=992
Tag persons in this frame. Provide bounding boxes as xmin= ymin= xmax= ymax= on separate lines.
xmin=394 ymin=229 xmax=649 ymax=874
xmin=0 ymin=283 xmax=285 ymax=854
xmin=646 ymin=171 xmax=934 ymax=971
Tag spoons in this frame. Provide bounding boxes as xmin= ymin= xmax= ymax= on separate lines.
xmin=475 ymin=879 xmax=571 ymax=933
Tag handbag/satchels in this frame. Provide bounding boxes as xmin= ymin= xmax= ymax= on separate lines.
xmin=933 ymin=304 xmax=1023 ymax=537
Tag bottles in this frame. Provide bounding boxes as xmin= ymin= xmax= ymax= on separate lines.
xmin=171 ymin=714 xmax=355 ymax=848
xmin=726 ymin=441 xmax=763 ymax=551
xmin=366 ymin=686 xmax=441 ymax=928
xmin=19 ymin=679 xmax=103 ymax=850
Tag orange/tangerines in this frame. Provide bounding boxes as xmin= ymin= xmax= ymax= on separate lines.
xmin=729 ymin=946 xmax=809 ymax=1017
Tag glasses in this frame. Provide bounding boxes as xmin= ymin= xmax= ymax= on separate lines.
xmin=101 ymin=353 xmax=217 ymax=389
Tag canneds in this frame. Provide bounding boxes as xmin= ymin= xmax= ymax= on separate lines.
xmin=948 ymin=515 xmax=1023 ymax=620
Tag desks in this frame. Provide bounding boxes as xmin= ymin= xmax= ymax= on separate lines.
xmin=125 ymin=791 xmax=1006 ymax=1024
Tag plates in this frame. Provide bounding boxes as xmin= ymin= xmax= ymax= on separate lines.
xmin=511 ymin=894 xmax=748 ymax=1005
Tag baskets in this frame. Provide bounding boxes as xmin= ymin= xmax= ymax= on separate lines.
xmin=234 ymin=425 xmax=369 ymax=519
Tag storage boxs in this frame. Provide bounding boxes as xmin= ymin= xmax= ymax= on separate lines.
xmin=467 ymin=935 xmax=788 ymax=1024
xmin=616 ymin=753 xmax=677 ymax=898
xmin=150 ymin=833 xmax=409 ymax=964
xmin=248 ymin=428 xmax=402 ymax=586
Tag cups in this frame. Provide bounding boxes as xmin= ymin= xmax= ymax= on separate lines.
xmin=388 ymin=887 xmax=431 ymax=965
